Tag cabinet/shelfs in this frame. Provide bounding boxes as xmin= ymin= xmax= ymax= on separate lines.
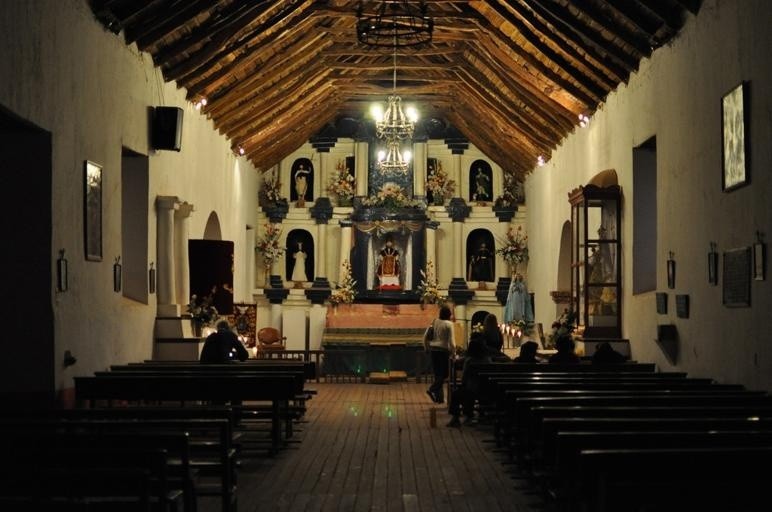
xmin=567 ymin=184 xmax=629 ymax=343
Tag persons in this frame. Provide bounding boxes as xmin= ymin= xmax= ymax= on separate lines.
xmin=198 ymin=321 xmax=249 ymax=428
xmin=591 ymin=343 xmax=625 ymax=370
xmin=514 ymin=341 xmax=540 ymax=362
xmin=377 ymin=234 xmax=401 ymax=287
xmin=549 ymin=333 xmax=581 ymax=362
xmin=473 ymin=240 xmax=492 ymax=281
xmin=295 ymin=164 xmax=312 ymax=200
xmin=291 ymin=240 xmax=309 ymax=281
xmin=472 ymin=167 xmax=490 ymax=201
xmin=423 ymin=306 xmax=457 ymax=403
xmin=446 ymin=314 xmax=511 ymax=426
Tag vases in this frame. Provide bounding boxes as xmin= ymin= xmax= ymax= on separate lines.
xmin=196 ymin=328 xmax=204 ymax=337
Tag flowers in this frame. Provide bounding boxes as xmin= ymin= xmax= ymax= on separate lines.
xmin=254 ymin=220 xmax=286 ymax=290
xmin=186 ymin=286 xmax=221 ymax=330
xmin=496 ymin=225 xmax=530 ymax=283
xmin=260 ymin=173 xmax=284 ymax=202
xmin=540 ymin=308 xmax=577 ymax=350
xmin=415 ymin=260 xmax=449 ymax=305
xmin=361 ymin=184 xmax=422 ymax=208
xmin=329 ymin=258 xmax=359 ymax=306
xmin=425 ymin=163 xmax=456 ymax=206
xmin=328 ymin=161 xmax=353 ymax=206
xmin=500 ymin=164 xmax=526 ymax=209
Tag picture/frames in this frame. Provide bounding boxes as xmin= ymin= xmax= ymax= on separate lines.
xmin=721 ymin=80 xmax=751 ymax=193
xmin=149 ymin=269 xmax=156 ymax=294
xmin=58 ymin=159 xmax=121 ymax=292
xmin=666 ymin=241 xmax=765 ymax=307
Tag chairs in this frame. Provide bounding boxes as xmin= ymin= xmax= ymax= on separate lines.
xmin=257 ymin=328 xmax=286 ymax=359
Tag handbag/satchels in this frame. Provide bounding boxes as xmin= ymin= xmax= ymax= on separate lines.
xmin=426 ymin=325 xmax=435 ymax=341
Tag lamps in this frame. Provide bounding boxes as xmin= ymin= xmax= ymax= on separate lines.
xmin=356 ymin=1 xmax=434 ymax=177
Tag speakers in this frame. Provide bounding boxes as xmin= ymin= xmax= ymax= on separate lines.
xmin=188 ymin=239 xmax=233 ymax=315
xmin=154 ymin=106 xmax=184 ymax=150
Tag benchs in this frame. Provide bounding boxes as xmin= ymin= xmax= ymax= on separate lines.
xmin=0 ymin=358 xmax=317 ymax=512
xmin=450 ymin=354 xmax=772 ymax=512
